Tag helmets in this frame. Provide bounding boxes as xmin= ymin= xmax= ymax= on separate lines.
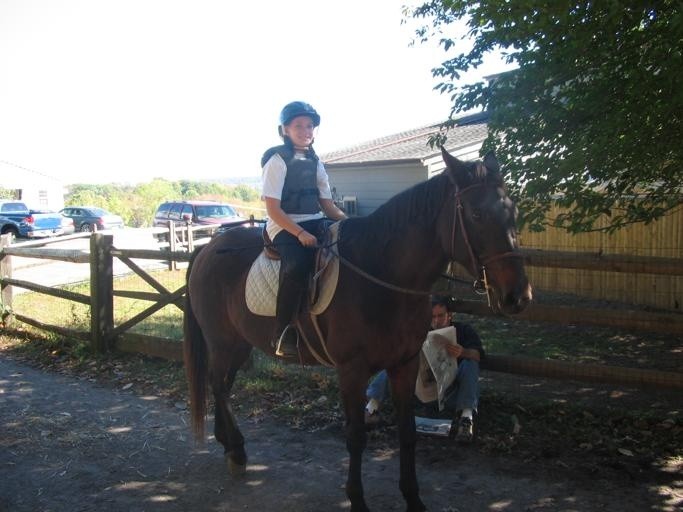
xmin=281 ymin=102 xmax=319 ymax=129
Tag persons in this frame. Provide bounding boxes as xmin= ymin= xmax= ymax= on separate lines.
xmin=363 ymin=295 xmax=485 ymax=444
xmin=260 ymin=101 xmax=350 ymax=358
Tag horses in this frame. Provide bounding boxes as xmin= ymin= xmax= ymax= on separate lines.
xmin=183 ymin=143 xmax=535 ymax=512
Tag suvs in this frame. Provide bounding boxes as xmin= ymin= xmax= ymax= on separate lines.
xmin=152 ymin=201 xmax=259 ymax=241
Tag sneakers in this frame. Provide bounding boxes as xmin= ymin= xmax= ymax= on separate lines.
xmin=276 ymin=338 xmax=297 ymax=354
xmin=456 ymin=416 xmax=474 ymax=441
xmin=343 ymin=408 xmax=381 ymax=427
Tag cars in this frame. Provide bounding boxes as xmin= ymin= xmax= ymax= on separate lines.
xmin=0 ymin=202 xmax=28 ymax=211
xmin=28 ymin=209 xmax=55 ymax=214
xmin=58 ymin=207 xmax=125 ymax=233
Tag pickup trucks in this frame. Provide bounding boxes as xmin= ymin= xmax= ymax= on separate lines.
xmin=0 ymin=212 xmax=63 ymax=239
